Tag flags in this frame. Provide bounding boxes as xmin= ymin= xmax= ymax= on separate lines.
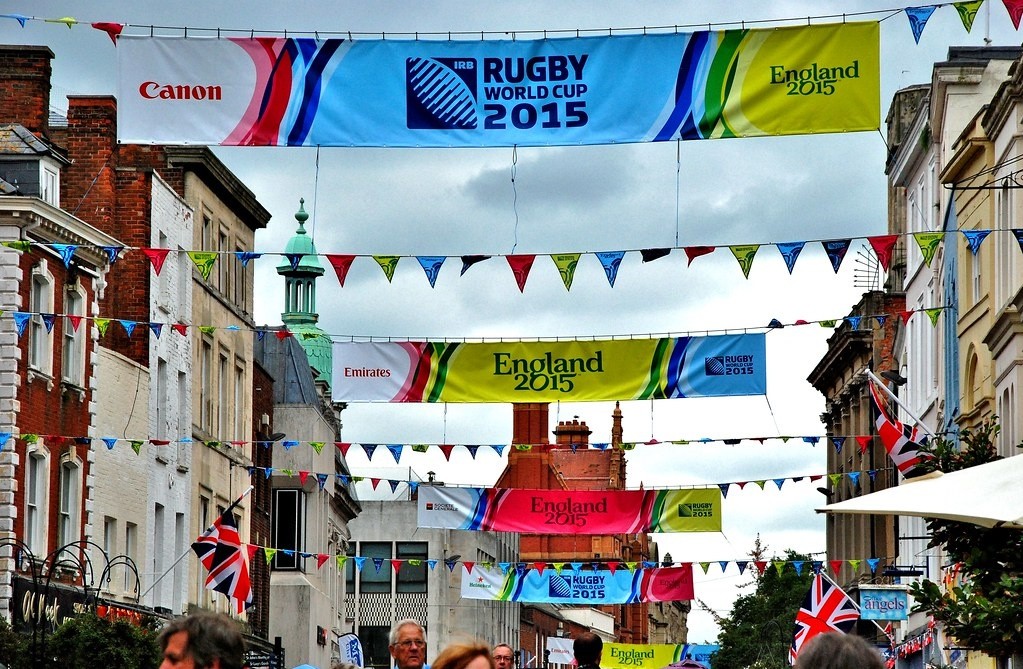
xmin=191 ymin=494 xmax=254 ymax=613
xmin=869 ymin=382 xmax=939 ymax=481
xmin=787 ymin=574 xmax=861 ymax=666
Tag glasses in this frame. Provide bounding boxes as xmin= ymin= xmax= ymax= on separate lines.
xmin=493 ymin=656 xmax=513 ymax=662
xmin=392 ymin=640 xmax=423 ymax=647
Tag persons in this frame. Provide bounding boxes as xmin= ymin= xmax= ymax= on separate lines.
xmin=388 ymin=619 xmax=430 ymax=669
xmin=155 ymin=609 xmax=245 ymax=669
xmin=493 ymin=644 xmax=514 ymax=669
xmin=431 ymin=641 xmax=497 ymax=669
xmin=574 ymin=632 xmax=603 ymax=669
xmin=797 ymin=631 xmax=885 ymax=669
